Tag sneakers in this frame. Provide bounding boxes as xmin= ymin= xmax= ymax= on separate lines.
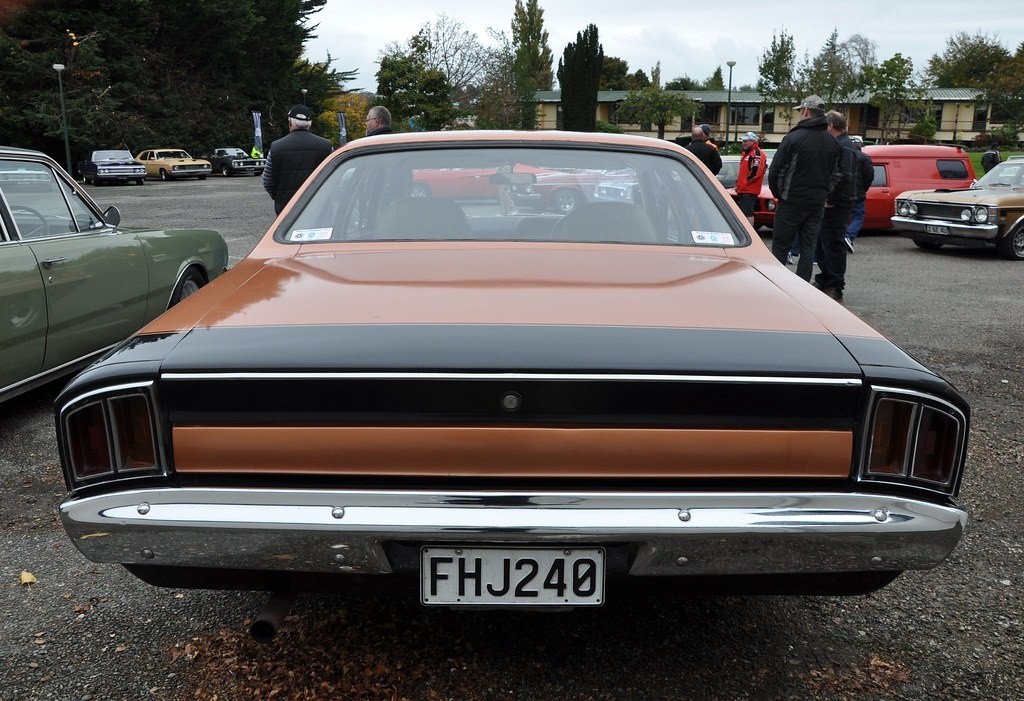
xmin=811 ymin=281 xmax=843 ymax=303
xmin=786 ymin=252 xmax=799 ymax=264
xmin=845 ymin=237 xmax=854 ymax=253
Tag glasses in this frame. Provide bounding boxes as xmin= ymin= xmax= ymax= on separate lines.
xmin=365 ymin=118 xmax=377 ymax=124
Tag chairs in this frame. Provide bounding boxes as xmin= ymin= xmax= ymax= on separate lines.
xmin=376 ymin=195 xmax=473 ymax=240
xmin=548 ymin=200 xmax=660 ymax=244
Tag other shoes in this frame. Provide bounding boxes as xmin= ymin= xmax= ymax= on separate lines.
xmin=496 ymin=211 xmax=519 ymax=217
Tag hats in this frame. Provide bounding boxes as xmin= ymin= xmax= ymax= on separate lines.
xmin=700 ymin=124 xmax=710 ymax=135
xmin=739 ymin=131 xmax=759 ymax=142
xmin=792 ymin=94 xmax=825 ymax=113
xmin=288 ymin=104 xmax=312 ymax=120
xmin=851 ymin=135 xmax=865 ymax=147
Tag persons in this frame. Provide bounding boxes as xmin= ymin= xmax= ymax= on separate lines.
xmin=736 ymin=131 xmax=767 ymax=228
xmin=261 ymin=105 xmax=334 ymax=217
xmin=813 ymin=110 xmax=858 ymax=303
xmin=981 ymin=142 xmax=1001 ymax=174
xmin=497 ymin=162 xmax=517 ymax=216
xmin=845 ymin=135 xmax=874 ymax=254
xmin=768 ymin=96 xmax=846 ymax=285
xmin=685 ymin=128 xmax=722 ymax=177
xmin=700 ymin=124 xmax=718 ymax=150
xmin=356 ymin=106 xmax=413 ymax=232
xmin=786 ymin=232 xmax=819 ymax=265
